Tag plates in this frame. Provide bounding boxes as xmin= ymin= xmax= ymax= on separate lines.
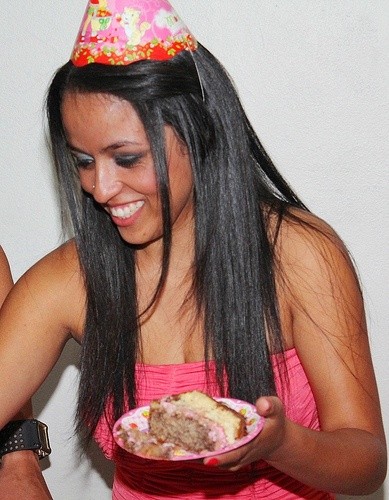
xmin=112 ymin=398 xmax=265 ymax=461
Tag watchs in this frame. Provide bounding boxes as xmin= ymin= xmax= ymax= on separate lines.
xmin=1 ymin=417 xmax=52 ymax=459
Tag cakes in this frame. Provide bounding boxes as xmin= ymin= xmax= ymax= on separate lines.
xmin=148 ymin=390 xmax=249 ymax=454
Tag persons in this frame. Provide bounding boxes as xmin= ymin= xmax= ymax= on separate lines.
xmin=0 ymin=1 xmax=387 ymax=500
xmin=0 ymin=247 xmax=53 ymax=500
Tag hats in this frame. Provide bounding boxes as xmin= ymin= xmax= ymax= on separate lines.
xmin=70 ymin=0 xmax=200 ymax=68
